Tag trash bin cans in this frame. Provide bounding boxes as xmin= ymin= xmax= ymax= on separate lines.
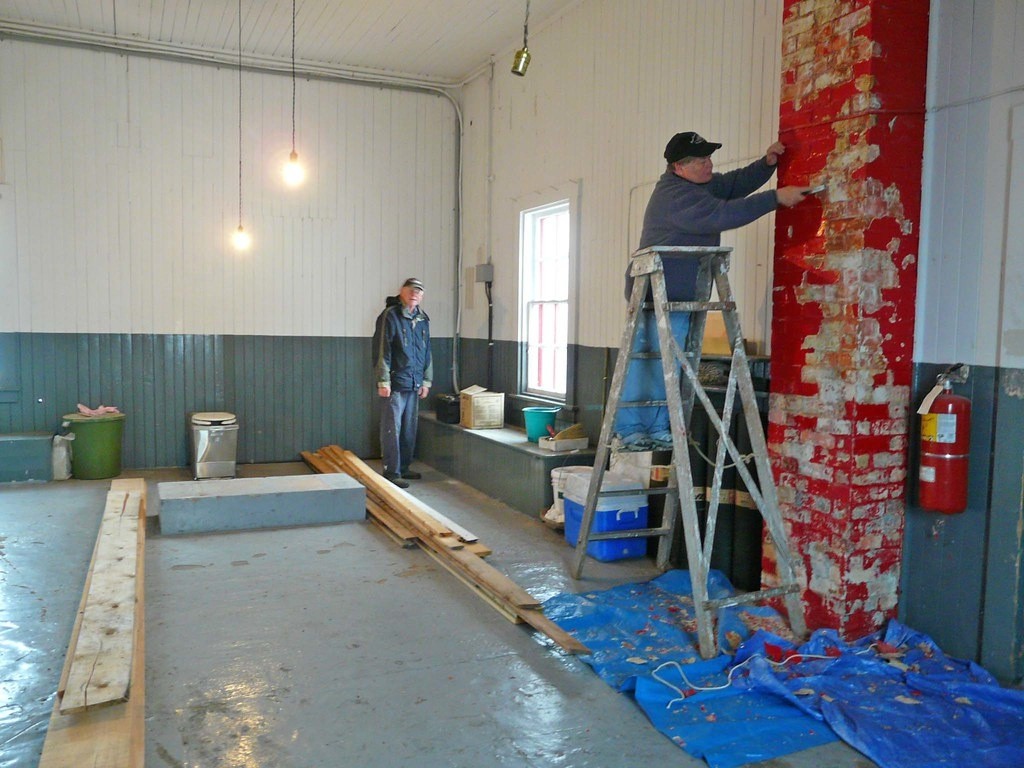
xmin=61 ymin=412 xmax=126 ymax=480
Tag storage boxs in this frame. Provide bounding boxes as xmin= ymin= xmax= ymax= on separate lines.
xmin=562 ymin=471 xmax=649 ymax=563
xmin=435 ymin=394 xmax=460 ymax=423
xmin=539 ymin=436 xmax=590 ymax=451
xmin=460 ymin=384 xmax=505 ymax=430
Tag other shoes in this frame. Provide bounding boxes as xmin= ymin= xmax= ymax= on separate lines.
xmin=390 ymin=478 xmax=409 ymax=488
xmin=402 ymin=471 xmax=421 ymax=479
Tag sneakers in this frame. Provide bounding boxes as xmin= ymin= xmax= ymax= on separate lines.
xmin=654 ymin=432 xmax=690 ymax=447
xmin=616 ymin=436 xmax=656 ymax=452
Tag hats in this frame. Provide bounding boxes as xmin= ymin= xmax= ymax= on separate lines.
xmin=403 ymin=278 xmax=425 ymax=291
xmin=664 ymin=131 xmax=722 ymax=163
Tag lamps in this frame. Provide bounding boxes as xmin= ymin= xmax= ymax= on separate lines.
xmin=512 ymin=47 xmax=530 ymax=77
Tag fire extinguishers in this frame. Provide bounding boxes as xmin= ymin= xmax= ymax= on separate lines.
xmin=919 ymin=363 xmax=971 ymax=515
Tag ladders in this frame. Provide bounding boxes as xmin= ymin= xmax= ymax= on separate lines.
xmin=568 ymin=245 xmax=810 ymax=658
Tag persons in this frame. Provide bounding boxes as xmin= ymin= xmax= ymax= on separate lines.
xmin=612 ymin=130 xmax=816 ymax=452
xmin=372 ymin=278 xmax=434 ymax=489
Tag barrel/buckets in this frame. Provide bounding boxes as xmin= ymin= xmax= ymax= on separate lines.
xmin=524 ymin=405 xmax=561 ymax=442
xmin=63 ymin=412 xmax=126 ymax=480
xmin=551 ymin=466 xmax=594 ymax=515
